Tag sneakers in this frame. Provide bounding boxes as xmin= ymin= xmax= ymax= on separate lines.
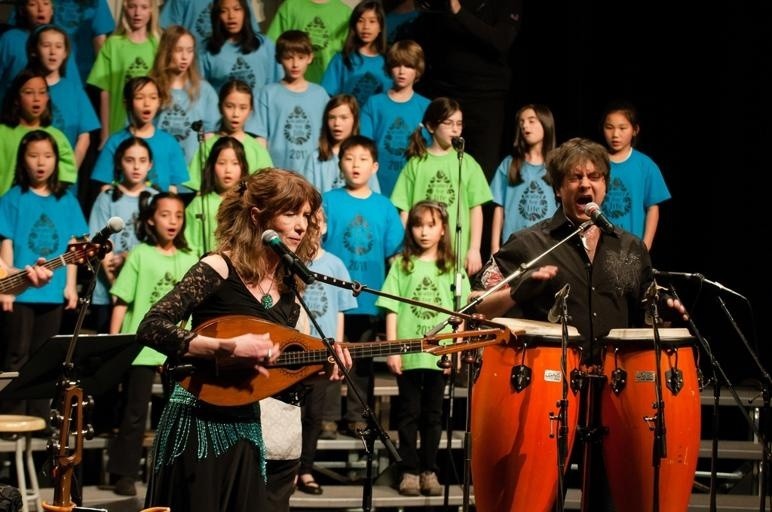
xmin=115 ymin=476 xmax=136 ymax=496
xmin=421 ymin=471 xmax=443 ymax=496
xmin=348 ymin=422 xmax=368 ymax=436
xmin=399 ymin=473 xmax=421 ymax=495
xmin=321 ymin=419 xmax=338 ymax=440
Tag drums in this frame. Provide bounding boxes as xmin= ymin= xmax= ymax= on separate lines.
xmin=469 ymin=317 xmax=583 ymax=511
xmin=602 ymin=327 xmax=702 ymax=512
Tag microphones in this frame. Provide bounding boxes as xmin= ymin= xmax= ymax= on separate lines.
xmin=645 ymin=281 xmax=658 ymax=326
xmin=548 ymin=283 xmax=570 ymax=324
xmin=260 ymin=230 xmax=314 ymax=284
xmin=85 ymin=217 xmax=124 ymax=248
xmin=190 ymin=120 xmax=204 ymax=131
xmin=450 ymin=135 xmax=464 ymax=152
xmin=585 ymin=201 xmax=616 ymax=236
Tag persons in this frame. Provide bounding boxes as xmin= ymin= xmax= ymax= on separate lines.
xmin=136 ymin=167 xmax=352 ymax=512
xmin=467 ymin=138 xmax=689 ymax=512
xmin=0 ymin=0 xmax=671 ymax=496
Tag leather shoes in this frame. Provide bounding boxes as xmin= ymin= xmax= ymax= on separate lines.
xmin=298 ymin=476 xmax=323 ymax=493
